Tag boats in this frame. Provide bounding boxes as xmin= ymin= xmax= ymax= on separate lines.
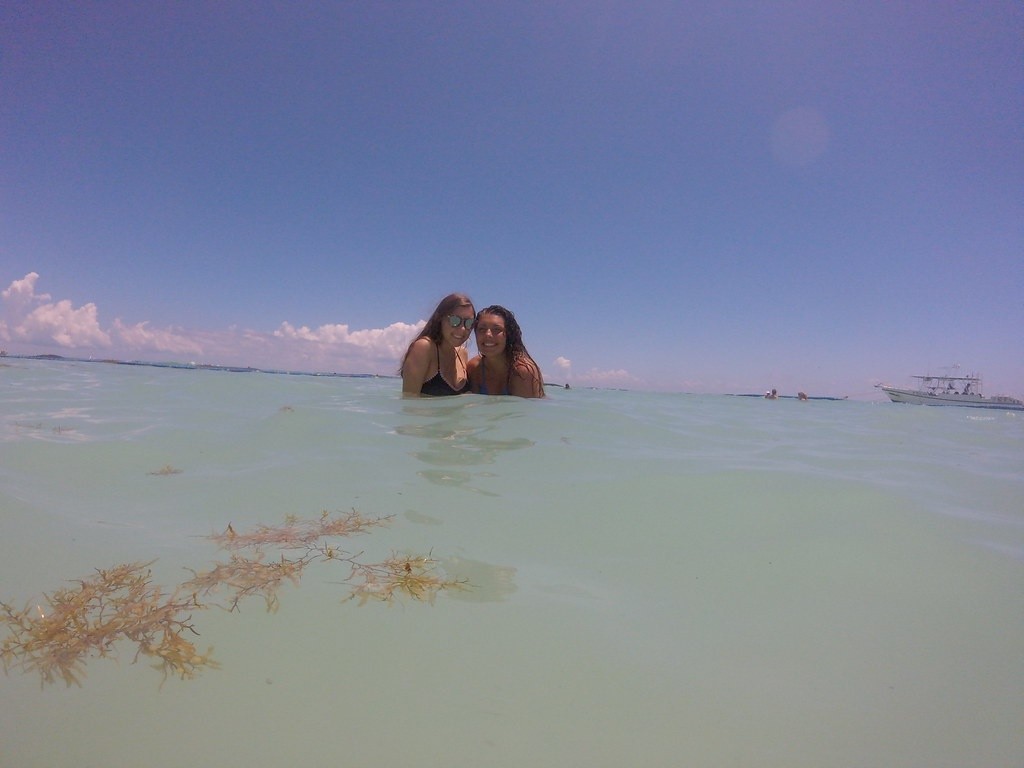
xmin=874 ymin=373 xmax=1024 ymax=411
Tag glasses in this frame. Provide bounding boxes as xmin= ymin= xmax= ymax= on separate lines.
xmin=446 ymin=314 xmax=478 ymax=329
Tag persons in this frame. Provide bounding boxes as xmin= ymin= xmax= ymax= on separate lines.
xmin=798 ymin=392 xmax=807 ymax=400
xmin=401 ymin=293 xmax=476 ymax=396
xmin=965 ymin=383 xmax=970 ymax=395
xmin=468 ymin=305 xmax=544 ymax=398
xmin=566 ymin=384 xmax=569 ymax=388
xmin=765 ymin=389 xmax=777 ymax=399
